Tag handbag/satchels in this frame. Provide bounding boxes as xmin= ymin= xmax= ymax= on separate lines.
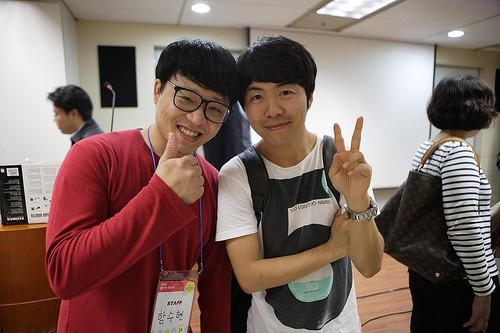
xmin=375 ymin=136 xmax=480 ymax=286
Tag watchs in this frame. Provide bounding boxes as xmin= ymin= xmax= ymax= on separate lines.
xmin=343 ymin=197 xmax=378 ymax=223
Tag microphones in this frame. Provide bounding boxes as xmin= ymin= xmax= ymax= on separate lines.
xmin=105 ymin=82 xmax=116 ymax=132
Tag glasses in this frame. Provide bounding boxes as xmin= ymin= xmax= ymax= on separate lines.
xmin=168 ymin=80 xmax=235 ymax=124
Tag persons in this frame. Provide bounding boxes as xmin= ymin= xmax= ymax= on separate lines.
xmin=45 ymin=39 xmax=237 ymax=333
xmin=409 ymin=75 xmax=500 ymax=333
xmin=47 ymin=85 xmax=104 ymax=145
xmin=214 ymin=35 xmax=385 ymax=333
